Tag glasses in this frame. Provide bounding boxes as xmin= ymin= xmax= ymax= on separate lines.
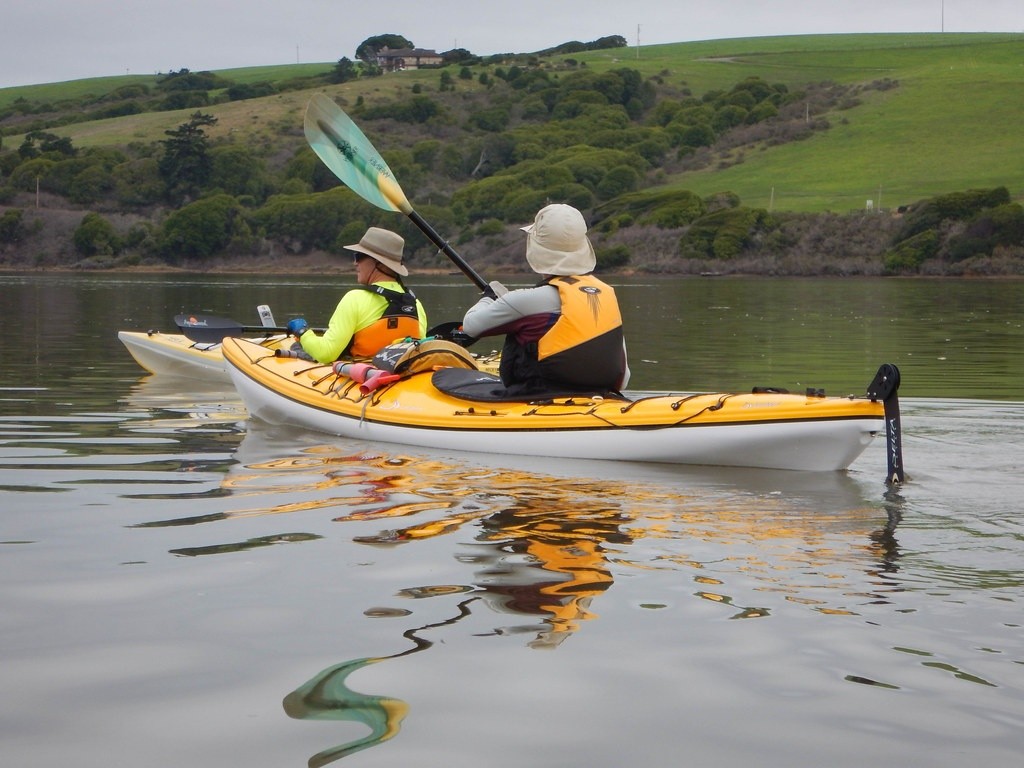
xmin=352 ymin=252 xmax=370 ymax=263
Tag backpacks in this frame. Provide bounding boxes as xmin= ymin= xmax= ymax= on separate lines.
xmin=372 ymin=337 xmax=478 ymax=370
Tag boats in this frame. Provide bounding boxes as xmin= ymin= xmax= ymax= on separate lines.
xmin=119 ymin=332 xmax=501 ymax=389
xmin=219 ymin=335 xmax=905 ymax=486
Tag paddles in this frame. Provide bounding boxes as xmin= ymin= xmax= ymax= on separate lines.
xmin=299 ymin=93 xmax=496 ymax=297
xmin=173 ymin=308 xmax=482 ymax=348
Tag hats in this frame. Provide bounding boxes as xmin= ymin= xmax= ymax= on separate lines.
xmin=342 ymin=226 xmax=411 ymax=277
xmin=521 ymin=204 xmax=597 ymax=277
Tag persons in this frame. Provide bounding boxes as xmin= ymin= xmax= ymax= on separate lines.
xmin=287 ymin=227 xmax=427 ymax=363
xmin=464 ymin=203 xmax=631 ymax=392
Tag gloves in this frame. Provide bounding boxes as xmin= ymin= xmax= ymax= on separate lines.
xmin=286 ymin=317 xmax=309 ymax=335
xmin=488 ymin=280 xmax=508 ymax=298
